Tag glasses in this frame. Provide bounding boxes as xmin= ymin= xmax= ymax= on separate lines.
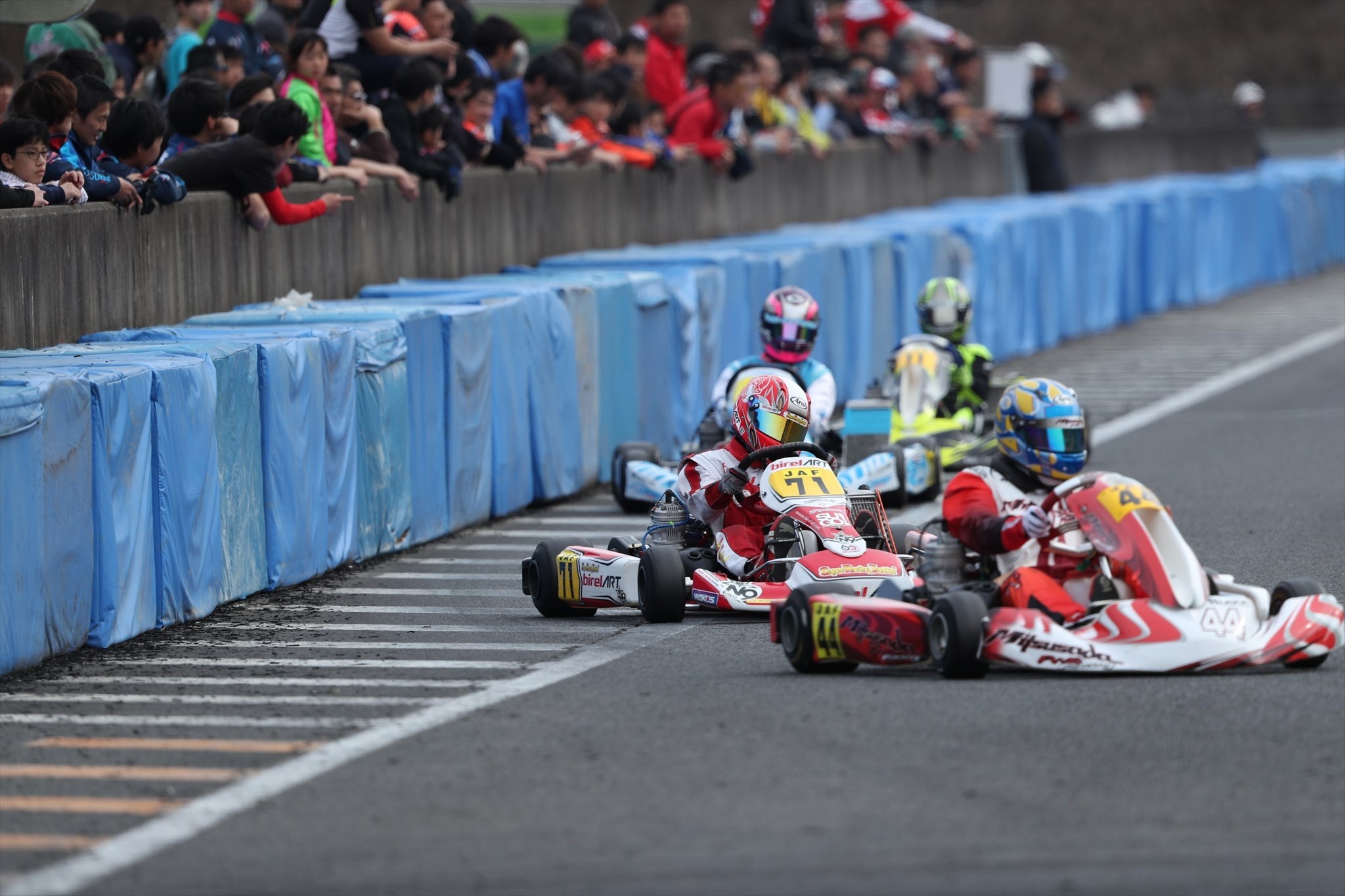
xmin=16 ymin=146 xmax=51 ymax=160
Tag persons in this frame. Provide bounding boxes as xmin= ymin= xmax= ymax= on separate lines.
xmin=945 ymin=379 xmax=1218 ymax=632
xmin=879 ymin=279 xmax=990 ymax=436
xmin=712 ymin=287 xmax=834 ymax=455
xmin=0 ymin=0 xmax=1068 ymax=230
xmin=1080 ymin=84 xmax=1160 ymax=131
xmin=675 ymin=367 xmax=882 ymax=582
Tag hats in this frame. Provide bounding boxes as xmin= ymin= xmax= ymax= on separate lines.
xmin=1016 ymin=42 xmax=1051 ymax=68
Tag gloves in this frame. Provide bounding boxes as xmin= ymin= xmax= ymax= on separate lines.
xmin=825 ymin=453 xmax=839 ymax=476
xmin=1001 ymin=506 xmax=1050 ymax=551
xmin=717 ymin=466 xmax=749 ymax=494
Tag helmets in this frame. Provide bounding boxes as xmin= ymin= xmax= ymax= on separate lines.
xmin=916 ymin=276 xmax=973 ymax=343
xmin=996 ymin=377 xmax=1087 ymax=480
xmin=759 ymin=286 xmax=819 ymax=363
xmin=731 ymin=375 xmax=811 ymax=462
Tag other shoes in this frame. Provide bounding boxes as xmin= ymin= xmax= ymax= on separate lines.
xmin=1207 ymin=574 xmax=1220 ymax=596
xmin=1089 ymin=575 xmax=1119 ymax=615
xmin=752 ymin=514 xmax=796 ymax=582
xmin=854 ymin=509 xmax=878 ymax=549
